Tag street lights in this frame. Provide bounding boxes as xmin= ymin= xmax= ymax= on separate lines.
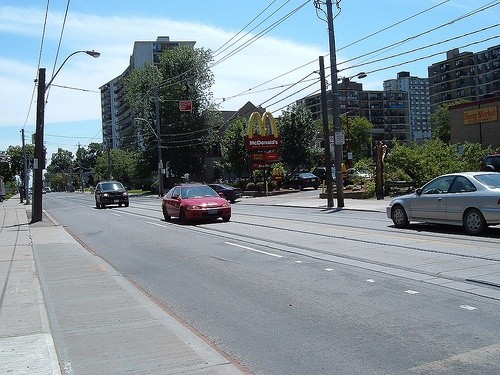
xmin=338 ymin=72 xmax=367 ymax=169
xmin=134 ymin=118 xmax=164 ymax=198
xmin=32 ymin=50 xmax=100 ymax=222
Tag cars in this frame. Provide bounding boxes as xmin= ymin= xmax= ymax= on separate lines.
xmin=161 ymin=184 xmax=232 ymax=225
xmin=281 ymin=167 xmax=373 ymax=191
xmin=386 ymin=172 xmax=500 ymax=235
xmin=28 ymin=187 xmax=51 ymax=195
xmin=208 ymin=184 xmax=242 ymax=202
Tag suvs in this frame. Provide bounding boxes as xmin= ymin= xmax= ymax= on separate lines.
xmin=94 ymin=181 xmax=129 ymax=209
